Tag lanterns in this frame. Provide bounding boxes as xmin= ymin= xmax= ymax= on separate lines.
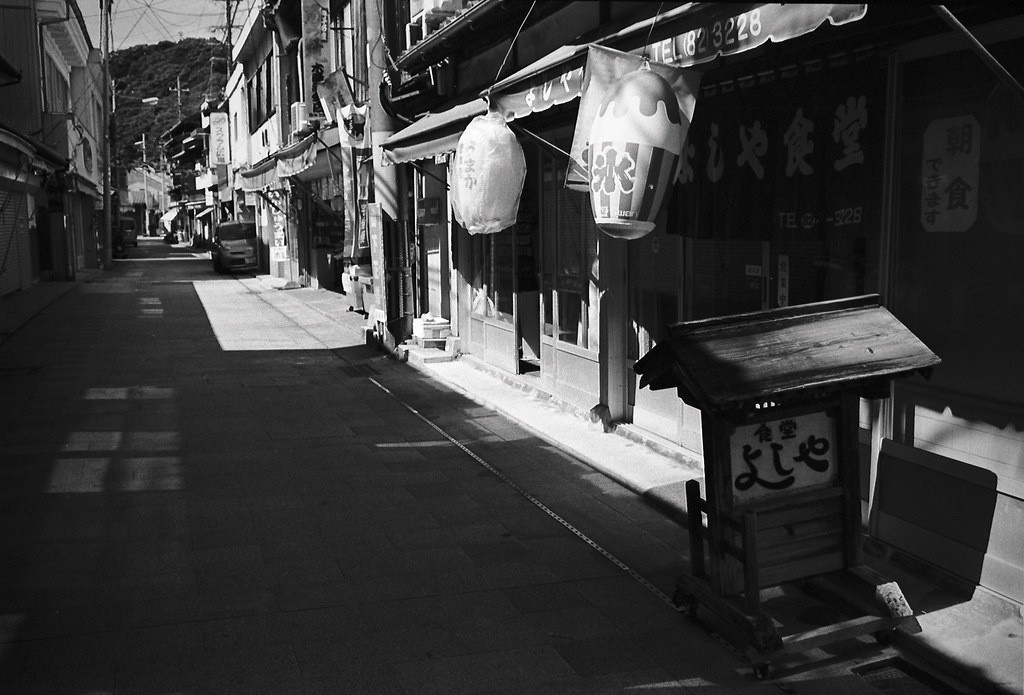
xmin=449 ymin=111 xmax=529 ymax=236
xmin=584 ymin=70 xmax=684 ymax=238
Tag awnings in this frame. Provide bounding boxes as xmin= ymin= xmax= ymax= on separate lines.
xmin=478 ymin=0 xmax=1023 ymax=163
xmin=160 ymin=207 xmax=183 ymax=222
xmin=196 ymin=207 xmax=216 ymax=217
xmin=241 ymin=127 xmax=345 ymax=221
xmin=376 ymin=97 xmax=490 ymax=189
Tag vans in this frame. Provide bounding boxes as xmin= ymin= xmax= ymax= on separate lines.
xmin=120 ymin=216 xmax=138 ymax=247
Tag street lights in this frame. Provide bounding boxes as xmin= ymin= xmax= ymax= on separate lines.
xmin=134 ymin=133 xmax=151 ymax=236
xmin=104 ymin=96 xmax=159 ymax=271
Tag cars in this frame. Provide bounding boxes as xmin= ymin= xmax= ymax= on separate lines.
xmin=211 ymin=220 xmax=258 ymax=274
